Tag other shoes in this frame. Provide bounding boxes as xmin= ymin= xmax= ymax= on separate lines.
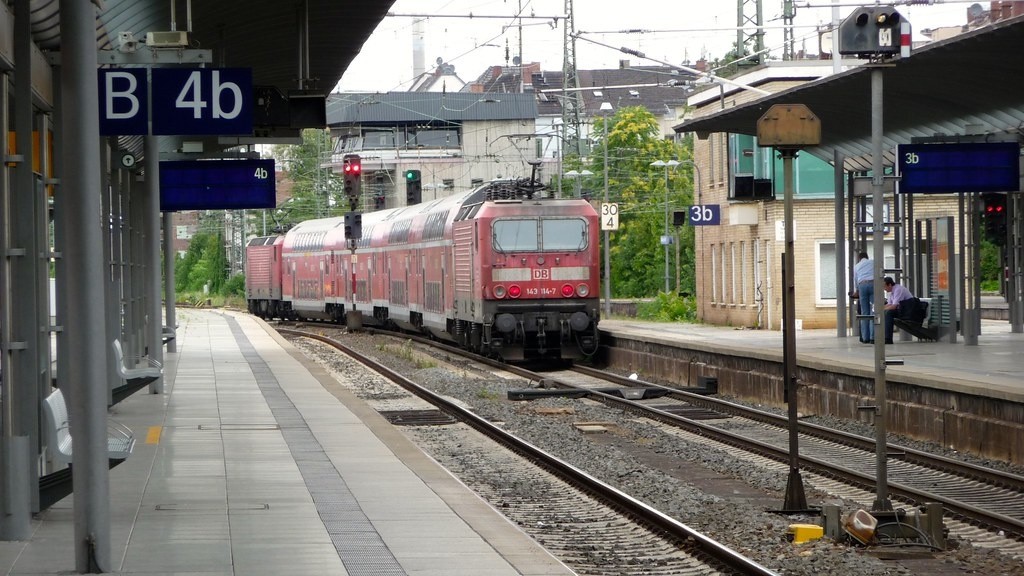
xmin=863 ymin=339 xmax=868 ymax=343
xmin=885 ymin=340 xmax=893 ymax=344
xmin=870 ymin=340 xmax=874 ymax=344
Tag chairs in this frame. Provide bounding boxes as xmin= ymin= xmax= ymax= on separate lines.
xmin=145 ymin=315 xmax=176 ymax=356
xmin=108 ymin=339 xmax=163 ymax=406
xmin=40 ymin=390 xmax=136 ymax=514
xmin=892 ymin=297 xmax=937 ymax=340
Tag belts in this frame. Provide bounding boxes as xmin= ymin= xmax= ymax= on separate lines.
xmin=860 ymin=280 xmax=873 ymax=284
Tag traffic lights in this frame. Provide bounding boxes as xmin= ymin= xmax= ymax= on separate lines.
xmin=343 ymin=212 xmax=362 ymax=239
xmin=984 ymin=191 xmax=1009 ymax=247
xmin=342 ymin=154 xmax=361 ymax=196
xmin=406 ymin=169 xmax=422 ymax=206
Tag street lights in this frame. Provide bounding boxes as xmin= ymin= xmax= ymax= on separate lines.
xmin=650 ymin=159 xmax=681 ymax=297
xmin=599 ymin=101 xmax=615 ymax=321
xmin=565 ymin=170 xmax=594 ymax=197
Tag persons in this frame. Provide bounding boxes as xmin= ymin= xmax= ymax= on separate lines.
xmin=852 ymin=253 xmax=875 ymax=344
xmin=883 ymin=277 xmax=914 ymax=344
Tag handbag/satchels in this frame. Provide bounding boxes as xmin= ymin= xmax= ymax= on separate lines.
xmin=897 ymin=298 xmax=921 ymax=319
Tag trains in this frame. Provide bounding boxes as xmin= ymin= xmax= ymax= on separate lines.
xmin=244 ymin=133 xmax=600 ymax=371
